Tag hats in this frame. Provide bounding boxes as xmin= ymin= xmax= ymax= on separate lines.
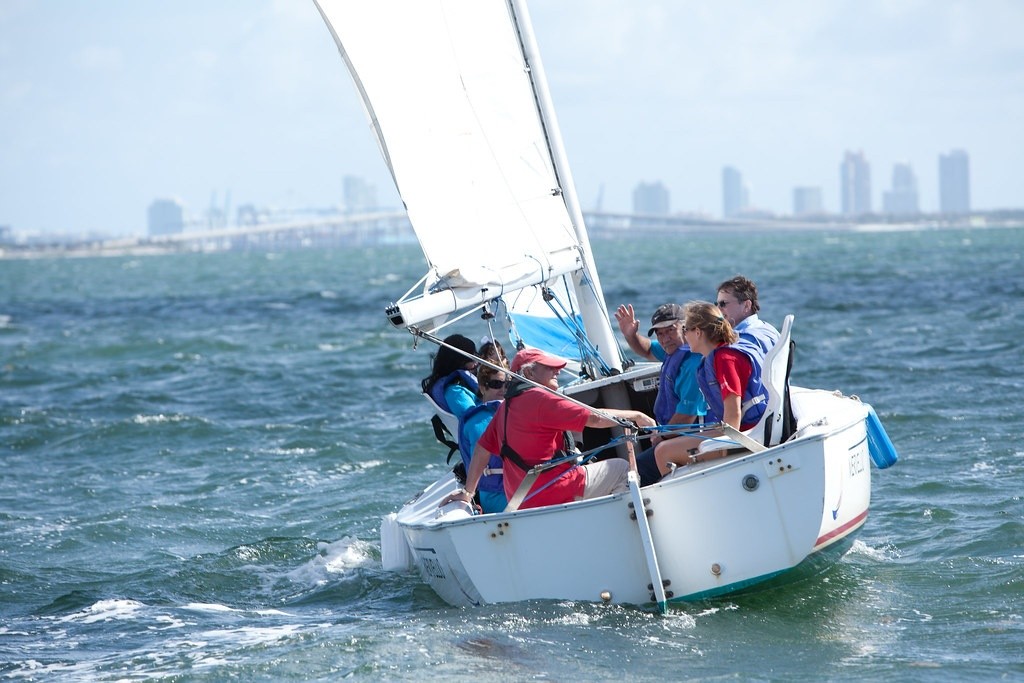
xmin=648 ymin=303 xmax=686 ymax=337
xmin=511 ymin=349 xmax=567 ymax=372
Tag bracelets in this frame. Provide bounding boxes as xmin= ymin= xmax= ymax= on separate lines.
xmin=462 ymin=486 xmax=475 ymax=497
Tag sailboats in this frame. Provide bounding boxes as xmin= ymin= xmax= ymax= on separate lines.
xmin=316 ymin=0 xmax=895 ymax=619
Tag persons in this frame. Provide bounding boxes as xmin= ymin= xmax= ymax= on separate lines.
xmin=615 ymin=303 xmax=722 ymax=488
xmin=458 ymin=358 xmax=509 ymax=513
xmin=438 ymin=348 xmax=659 ymax=510
xmin=425 ymin=333 xmax=483 ymax=446
xmin=654 ymin=303 xmax=784 ymax=478
xmin=714 ymin=276 xmax=781 ymax=354
xmin=479 ymin=339 xmax=509 ymax=365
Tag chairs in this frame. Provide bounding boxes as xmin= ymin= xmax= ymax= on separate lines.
xmin=660 ymin=314 xmax=797 ymax=482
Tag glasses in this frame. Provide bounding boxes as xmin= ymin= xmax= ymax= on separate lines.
xmin=482 ymin=378 xmax=511 ymax=390
xmin=713 ymin=299 xmax=748 ymax=307
xmin=463 ymin=351 xmax=480 ymax=362
xmin=682 ymin=326 xmax=698 ymax=334
